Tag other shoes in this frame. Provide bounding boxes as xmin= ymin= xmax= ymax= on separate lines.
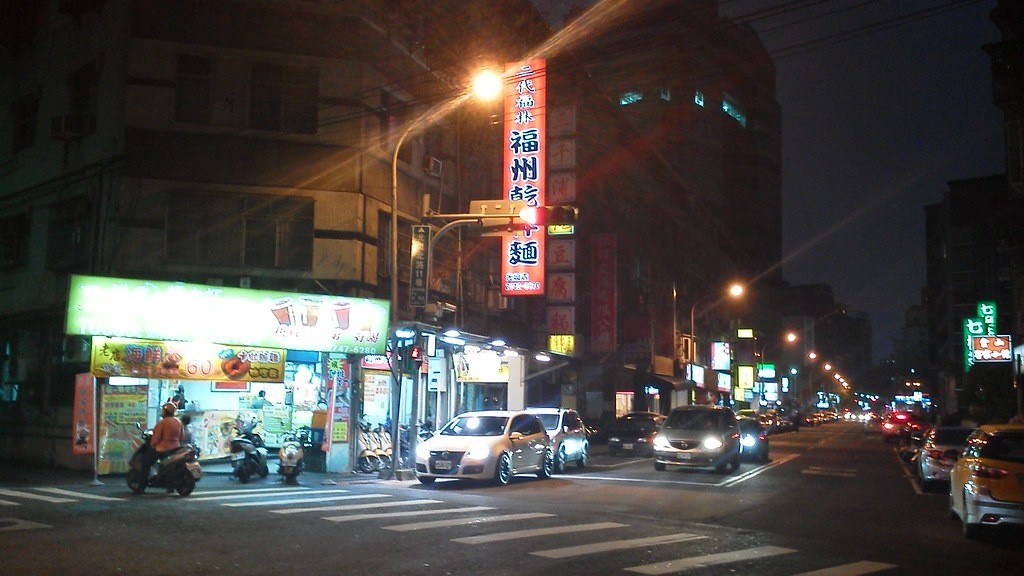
xmin=133 ymin=486 xmax=144 ymax=495
xmin=166 ymin=488 xmax=173 ymax=493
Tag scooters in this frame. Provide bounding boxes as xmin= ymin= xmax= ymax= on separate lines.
xmin=357 ymin=411 xmax=434 ymax=475
xmin=127 ymin=420 xmax=204 ymax=495
xmin=228 ymin=416 xmax=270 ymax=484
xmin=276 ymin=421 xmax=314 ymax=484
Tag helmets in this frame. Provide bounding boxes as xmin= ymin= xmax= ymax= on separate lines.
xmin=163 ymin=402 xmax=176 ymax=415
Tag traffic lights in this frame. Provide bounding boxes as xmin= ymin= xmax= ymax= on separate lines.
xmin=407 ymin=344 xmax=423 ymax=362
xmin=518 ymin=205 xmax=577 ymax=225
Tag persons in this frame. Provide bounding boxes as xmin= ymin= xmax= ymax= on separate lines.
xmin=134 ymin=402 xmax=185 ymax=494
xmin=167 ymin=385 xmax=188 ymax=409
xmin=704 ymin=417 xmax=716 ymax=429
xmin=250 ymin=390 xmax=273 ymax=409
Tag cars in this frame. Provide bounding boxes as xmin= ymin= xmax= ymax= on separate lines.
xmin=915 ymin=425 xmax=977 ymax=493
xmin=944 ymin=423 xmax=1024 ymax=539
xmin=524 ymin=406 xmax=590 ymax=472
xmin=736 ymin=409 xmax=842 ymax=436
xmin=652 ymin=404 xmax=742 ymax=475
xmin=414 ymin=409 xmax=555 ymax=486
xmin=737 ymin=415 xmax=770 ymax=463
xmin=844 ymin=411 xmax=935 ymax=464
xmin=608 ymin=411 xmax=668 ymax=457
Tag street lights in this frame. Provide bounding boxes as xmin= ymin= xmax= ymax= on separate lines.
xmin=688 ymin=285 xmax=743 ymax=406
xmin=387 ymin=70 xmax=502 ymax=324
xmin=761 ymin=334 xmax=796 ymax=370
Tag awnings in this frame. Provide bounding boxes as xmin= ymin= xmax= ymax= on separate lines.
xmin=647 ymin=373 xmax=697 ymax=391
